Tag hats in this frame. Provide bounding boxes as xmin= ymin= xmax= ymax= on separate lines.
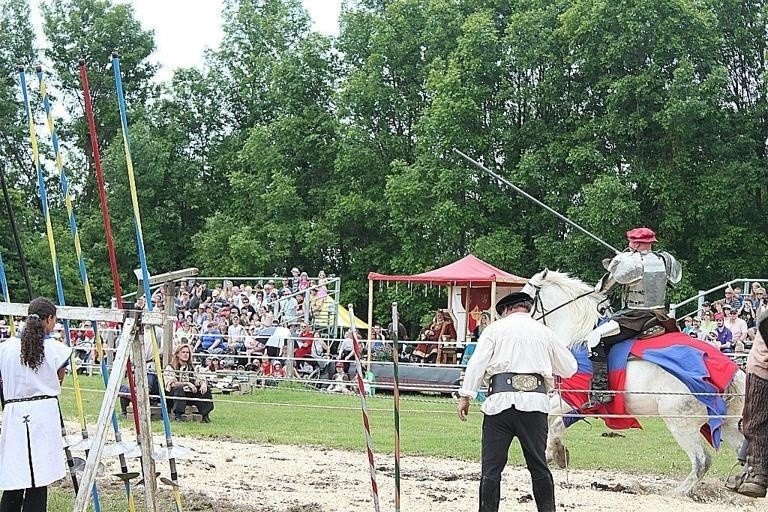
xmin=273 ymin=361 xmax=281 ymax=368
xmin=496 ymin=292 xmax=533 ymax=315
xmin=268 ymin=280 xmax=274 ymax=283
xmin=211 ymin=290 xmax=219 ymax=297
xmin=182 ymin=291 xmax=188 ymax=296
xmin=291 ymin=267 xmax=300 ymax=273
xmin=627 ymin=228 xmax=658 ymax=243
xmin=336 ymin=363 xmax=344 ymax=367
xmin=212 ymin=357 xmax=220 ymax=363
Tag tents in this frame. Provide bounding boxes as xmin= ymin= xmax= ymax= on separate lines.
xmin=367 ymin=253 xmax=532 ymax=374
xmin=311 ymin=295 xmax=368 ymax=347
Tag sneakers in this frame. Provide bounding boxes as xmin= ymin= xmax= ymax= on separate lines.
xmin=726 ymin=471 xmax=768 ymax=497
xmin=200 ymin=417 xmax=212 ymax=423
xmin=174 ymin=416 xmax=185 ymax=423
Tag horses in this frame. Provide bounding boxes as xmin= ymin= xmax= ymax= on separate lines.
xmin=517 ymin=265 xmax=748 ymax=494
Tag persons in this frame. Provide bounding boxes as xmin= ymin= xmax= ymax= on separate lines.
xmin=0 ymin=296 xmax=72 ymax=512
xmin=51 ymin=321 xmax=120 ymax=375
xmin=680 ymin=281 xmax=768 ymax=361
xmin=387 ymin=315 xmax=408 ymax=361
xmin=582 ymin=228 xmax=682 ymax=409
xmin=461 ymin=314 xmax=489 ymax=365
xmin=363 ymin=325 xmax=385 ymax=355
xmin=131 ymin=269 xmax=375 ymax=395
xmin=723 ymin=309 xmax=768 ymax=497
xmin=0 ymin=316 xmax=25 ymax=338
xmin=163 ymin=344 xmax=214 ymax=422
xmin=413 ymin=311 xmax=457 ymax=363
xmin=119 ymin=362 xmax=160 ymax=418
xmin=456 ymin=292 xmax=578 ymax=512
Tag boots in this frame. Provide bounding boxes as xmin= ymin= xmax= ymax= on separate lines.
xmin=581 ymin=361 xmax=612 ymax=408
